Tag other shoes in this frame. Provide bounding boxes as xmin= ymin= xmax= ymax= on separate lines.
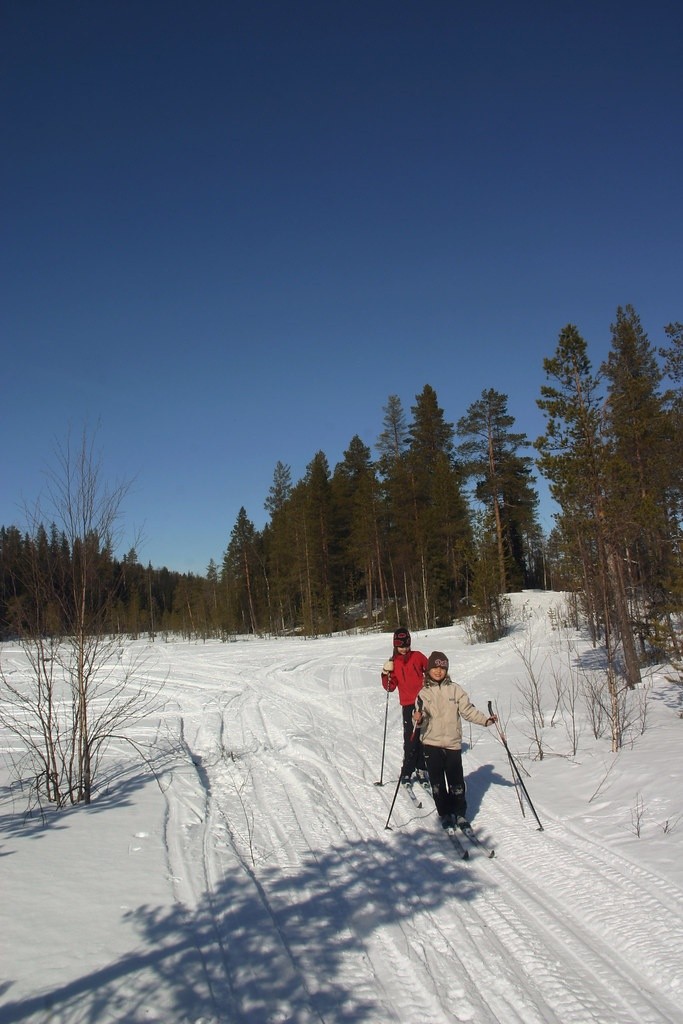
xmin=401 ymin=775 xmax=411 ymax=788
xmin=416 ymin=769 xmax=429 ymax=785
xmin=441 ymin=812 xmax=471 ymax=830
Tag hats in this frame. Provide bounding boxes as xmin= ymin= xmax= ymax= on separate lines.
xmin=393 ymin=627 xmax=412 ymax=647
xmin=427 ymin=650 xmax=449 ymax=670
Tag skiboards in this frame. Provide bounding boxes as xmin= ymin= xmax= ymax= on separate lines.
xmin=446 ymin=818 xmax=496 ymax=859
xmin=397 ymin=770 xmax=433 ymax=808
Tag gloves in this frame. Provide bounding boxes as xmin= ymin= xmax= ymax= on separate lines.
xmin=382 ymin=658 xmax=394 ymax=675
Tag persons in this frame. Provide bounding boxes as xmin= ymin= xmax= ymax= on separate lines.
xmin=381 ymin=630 xmax=435 ymax=787
xmin=412 ymin=652 xmax=498 ymax=834
